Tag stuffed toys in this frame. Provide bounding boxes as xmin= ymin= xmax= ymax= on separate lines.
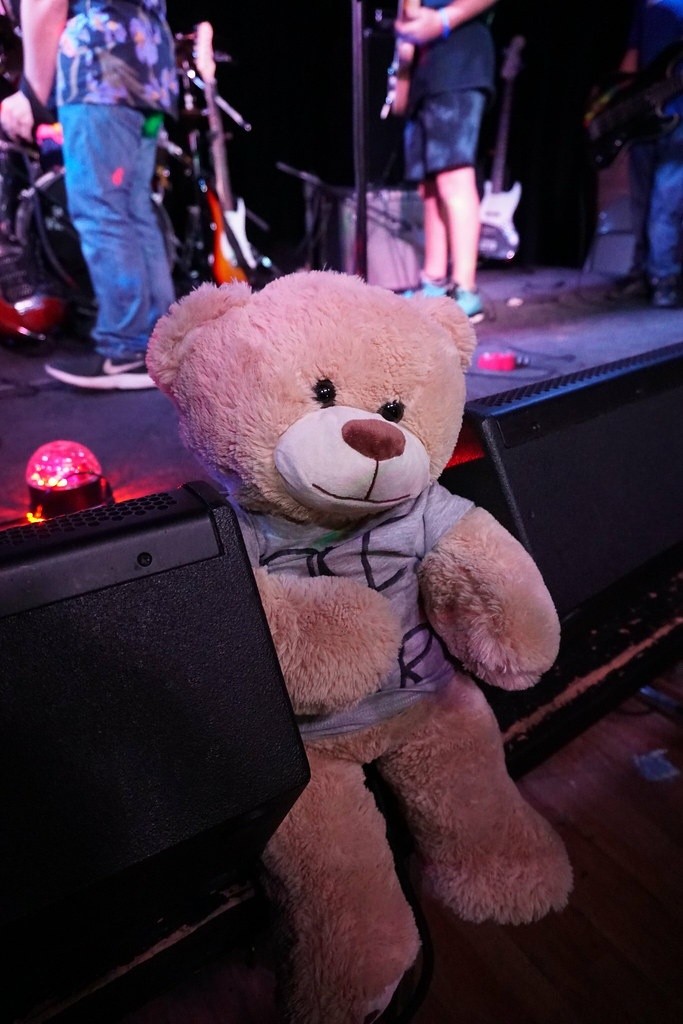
xmin=145 ymin=269 xmax=572 ymax=1024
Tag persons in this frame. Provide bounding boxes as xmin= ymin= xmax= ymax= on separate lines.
xmin=0 ymin=1 xmax=180 ymax=391
xmin=391 ymin=0 xmax=496 ymax=325
xmin=608 ymin=0 xmax=683 ymax=307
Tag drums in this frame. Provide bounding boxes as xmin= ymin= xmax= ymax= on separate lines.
xmin=0 ymin=138 xmax=43 ymax=268
xmin=15 ymin=139 xmax=216 ymax=318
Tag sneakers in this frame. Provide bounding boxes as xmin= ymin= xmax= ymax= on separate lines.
xmin=388 ymin=268 xmax=451 ymax=298
xmin=44 ymin=338 xmax=177 ymax=389
xmin=428 ymin=283 xmax=486 ymax=326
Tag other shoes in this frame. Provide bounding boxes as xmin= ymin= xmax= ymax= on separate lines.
xmin=654 ymin=275 xmax=683 ymax=308
xmin=556 ymin=258 xmax=652 ymax=308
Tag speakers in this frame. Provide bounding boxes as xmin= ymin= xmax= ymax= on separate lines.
xmin=320 ymin=187 xmax=427 ymax=292
xmin=438 ymin=340 xmax=683 ymax=623
xmin=0 ymin=481 xmax=313 ymax=989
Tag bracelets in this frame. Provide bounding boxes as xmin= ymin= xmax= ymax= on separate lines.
xmin=439 ymin=6 xmax=451 ymax=38
xmin=18 ymin=73 xmax=54 ymax=124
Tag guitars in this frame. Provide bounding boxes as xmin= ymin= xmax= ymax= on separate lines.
xmin=191 ymin=20 xmax=259 ymax=295
xmin=478 ymin=34 xmax=529 ymax=261
xmin=380 ymin=0 xmax=421 ymax=119
xmin=580 ymin=39 xmax=683 ymax=169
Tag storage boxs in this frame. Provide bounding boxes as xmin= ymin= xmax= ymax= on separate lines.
xmin=302 ymin=182 xmax=424 ymax=292
